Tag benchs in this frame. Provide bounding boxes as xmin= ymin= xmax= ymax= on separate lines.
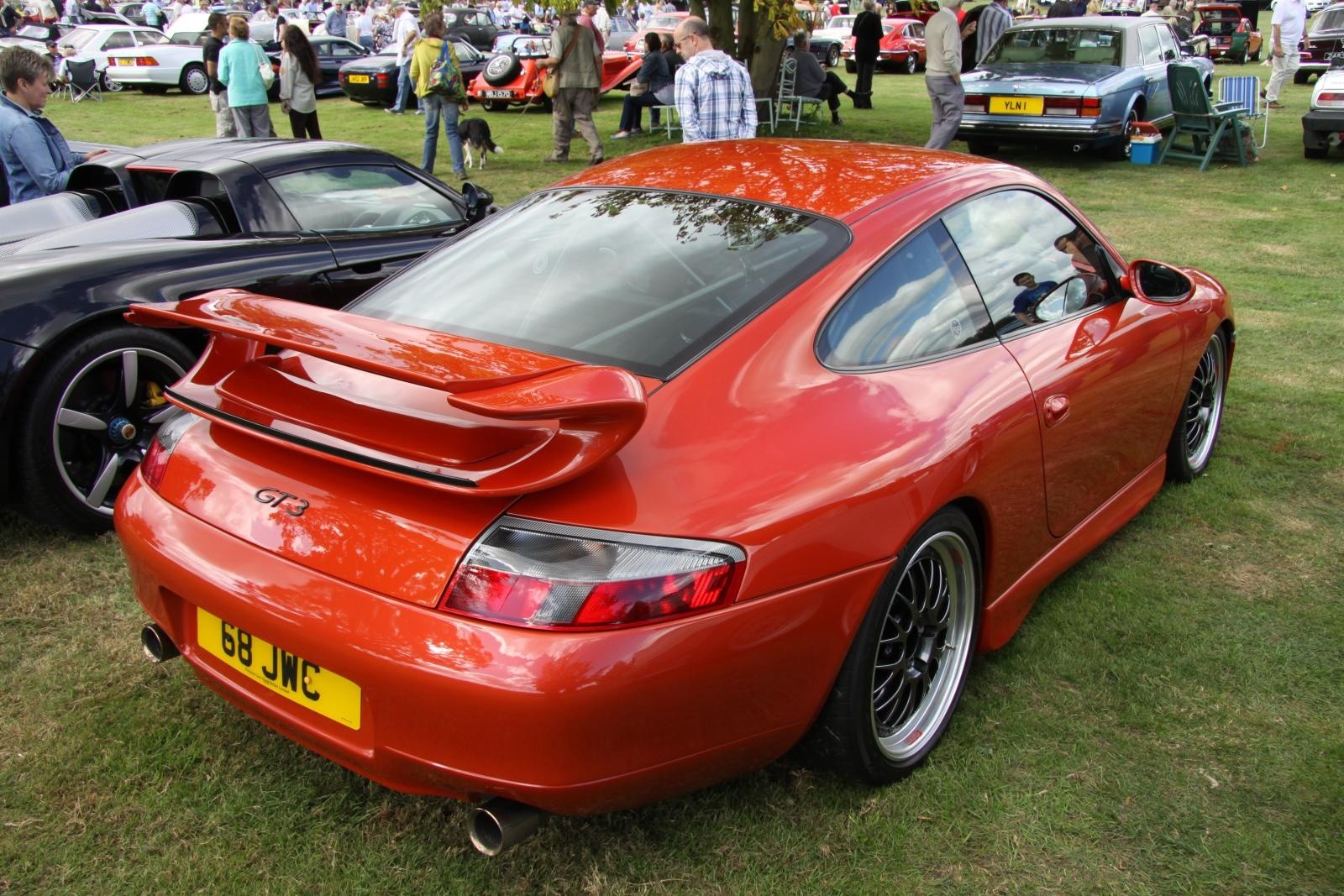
xmin=641 ymin=98 xmax=776 ymax=137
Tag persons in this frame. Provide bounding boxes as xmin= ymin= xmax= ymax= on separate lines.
xmin=0 ymin=44 xmax=116 ymax=207
xmin=526 ymin=6 xmax=608 ymax=165
xmin=404 ymin=9 xmax=472 ymax=184
xmin=0 ymin=0 xmax=1341 ymax=153
xmin=991 ymin=225 xmax=1121 ymax=330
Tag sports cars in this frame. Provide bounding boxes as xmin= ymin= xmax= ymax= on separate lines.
xmin=0 ymin=127 xmax=500 ymax=528
xmin=115 ymin=126 xmax=1237 ymax=874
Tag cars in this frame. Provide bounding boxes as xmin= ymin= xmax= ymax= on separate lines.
xmin=1193 ymin=3 xmax=1344 ymax=160
xmin=5 ymin=0 xmax=957 ymax=136
xmin=945 ymin=17 xmax=1215 ymax=154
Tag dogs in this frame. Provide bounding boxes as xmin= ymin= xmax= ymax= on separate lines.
xmin=458 ymin=118 xmax=503 ymax=170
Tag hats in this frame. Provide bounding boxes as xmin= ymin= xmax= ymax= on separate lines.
xmin=63 ymin=44 xmax=75 ymax=49
xmin=560 ymin=2 xmax=581 ymax=14
xmin=388 ymin=0 xmax=405 ymax=13
xmin=46 ymin=40 xmax=57 ymax=49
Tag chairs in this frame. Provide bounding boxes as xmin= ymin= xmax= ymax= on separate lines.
xmin=1158 ymin=62 xmax=1251 ymax=173
xmin=64 ymin=56 xmax=106 ymax=105
xmin=1036 ymin=38 xmax=1085 ymax=71
xmin=42 ymin=78 xmax=72 ymax=107
xmin=771 ymin=52 xmax=824 ymax=133
xmin=1212 ymin=74 xmax=1274 ymax=153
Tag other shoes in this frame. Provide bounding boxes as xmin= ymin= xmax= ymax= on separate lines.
xmin=544 ymin=156 xmax=569 ymax=164
xmin=854 ymin=91 xmax=873 ymax=105
xmin=1265 ymin=59 xmax=1273 ymax=66
xmin=454 ymin=172 xmax=468 ymax=180
xmin=384 ymin=108 xmax=405 ymax=115
xmin=587 ymin=156 xmax=604 ymax=167
xmin=411 ymin=109 xmax=426 ymax=115
xmin=571 ymin=129 xmax=584 ymax=138
xmin=1260 ymin=88 xmax=1267 ymax=100
xmin=1263 ymin=100 xmax=1285 ymax=108
xmin=630 ymin=127 xmax=642 ymax=135
xmin=831 ymin=118 xmax=844 ymax=126
xmin=610 ymin=130 xmax=631 ymax=140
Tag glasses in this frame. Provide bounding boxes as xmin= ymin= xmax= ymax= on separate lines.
xmin=336 ymin=4 xmax=342 ymax=6
xmin=202 ymin=4 xmax=207 ymax=6
xmin=674 ymin=32 xmax=697 ymax=49
xmin=50 ymin=45 xmax=57 ymax=48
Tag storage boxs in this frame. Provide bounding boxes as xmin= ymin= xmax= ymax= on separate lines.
xmin=1132 ymin=130 xmax=1161 ymax=166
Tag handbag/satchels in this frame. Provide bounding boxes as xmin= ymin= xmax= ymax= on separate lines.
xmin=258 ymin=61 xmax=276 ymax=90
xmin=427 ymin=60 xmax=464 ymax=103
xmin=629 ymin=78 xmax=649 ymax=97
xmin=542 ymin=67 xmax=560 ymax=98
